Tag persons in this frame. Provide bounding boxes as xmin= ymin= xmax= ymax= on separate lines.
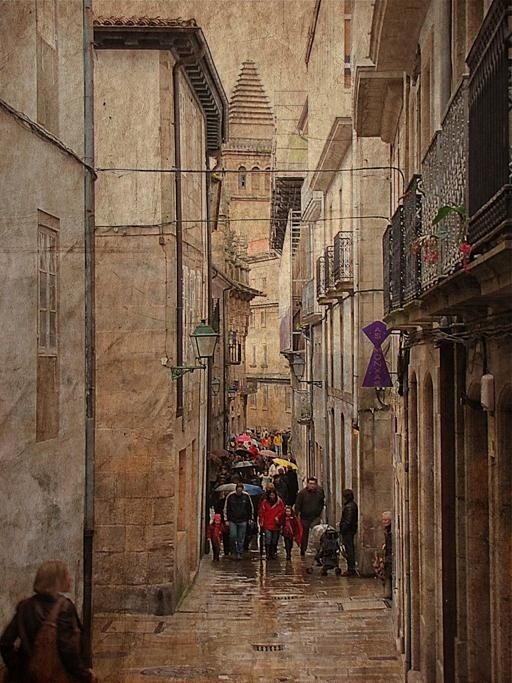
xmin=1 ymin=559 xmax=98 ymax=682
xmin=200 ymin=425 xmax=328 ymax=562
xmin=381 ymin=510 xmax=393 ymax=603
xmin=334 ymin=487 xmax=359 ymax=577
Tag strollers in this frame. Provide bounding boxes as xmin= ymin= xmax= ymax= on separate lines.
xmin=306 ymin=523 xmax=346 ymax=576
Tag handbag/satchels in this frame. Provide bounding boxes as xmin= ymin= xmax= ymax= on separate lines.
xmin=28 ymin=621 xmax=71 ymax=683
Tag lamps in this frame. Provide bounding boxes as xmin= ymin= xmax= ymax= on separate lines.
xmin=289 ymin=353 xmax=323 ymax=391
xmin=169 ymin=316 xmax=220 ymax=381
